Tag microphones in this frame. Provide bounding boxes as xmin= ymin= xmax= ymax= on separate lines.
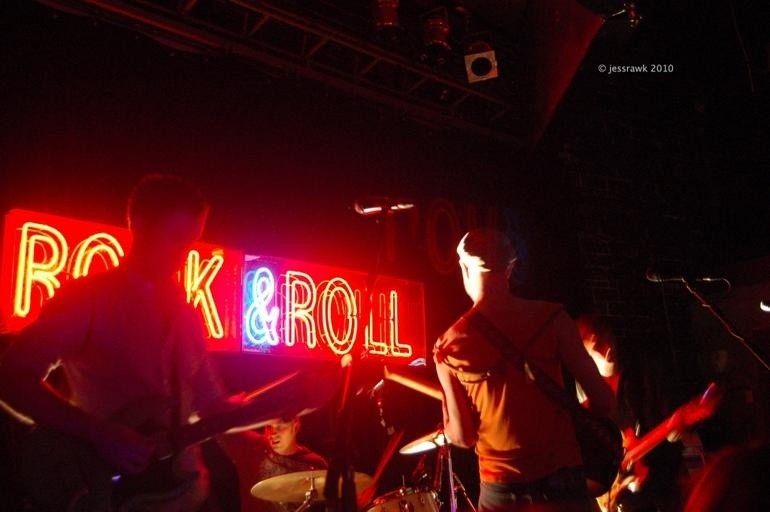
xmin=352 ymin=197 xmax=417 ymax=216
xmin=644 ymin=264 xmax=715 ymax=283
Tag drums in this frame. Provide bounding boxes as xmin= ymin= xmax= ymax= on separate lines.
xmin=361 ymin=485 xmax=444 ymax=511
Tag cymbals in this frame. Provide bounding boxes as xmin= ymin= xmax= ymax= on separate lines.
xmin=251 ymin=471 xmax=374 ymax=502
xmin=399 ymin=431 xmax=452 ymax=456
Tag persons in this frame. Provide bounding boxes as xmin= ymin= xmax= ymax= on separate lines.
xmin=431 ymin=221 xmax=619 ymax=511
xmin=574 ymin=309 xmax=702 ymax=512
xmin=681 ymin=444 xmax=770 ymax=512
xmin=1 ymin=169 xmax=257 ymax=512
xmin=215 ymin=414 xmax=328 ymax=511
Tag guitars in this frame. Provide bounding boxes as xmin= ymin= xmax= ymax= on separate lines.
xmin=595 ymin=382 xmax=722 ymax=512
xmin=383 ymin=363 xmax=622 ymax=496
xmin=19 ymin=366 xmax=339 ymax=512
